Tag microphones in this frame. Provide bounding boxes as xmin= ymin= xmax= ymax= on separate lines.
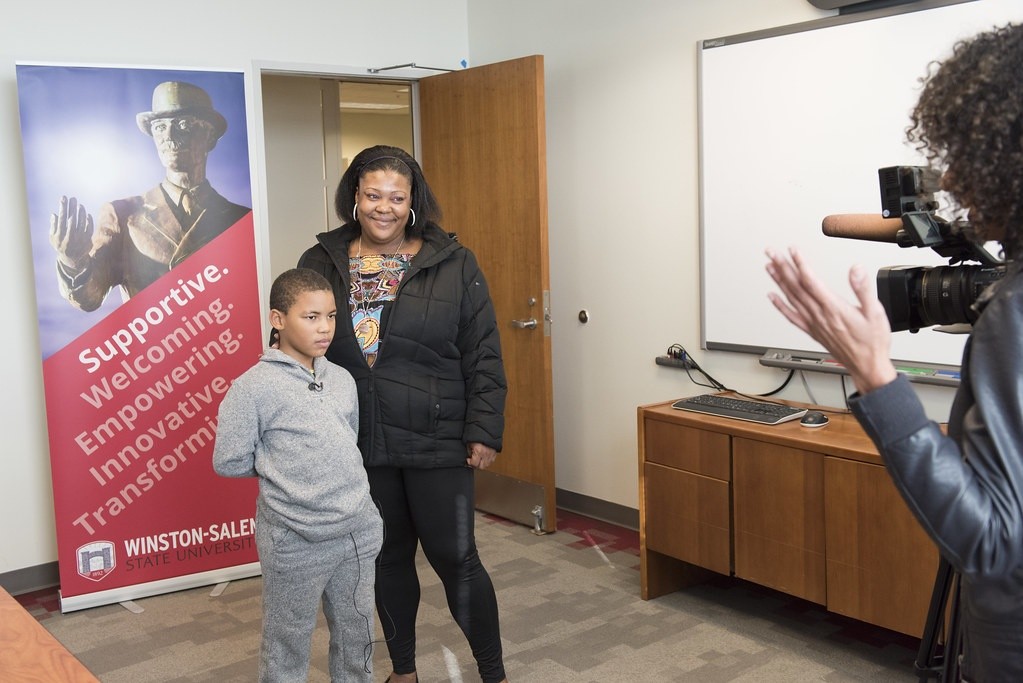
xmin=822 ymin=214 xmax=905 ymax=242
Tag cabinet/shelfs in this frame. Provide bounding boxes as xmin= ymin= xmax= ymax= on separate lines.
xmin=637 ymin=392 xmax=957 ymax=644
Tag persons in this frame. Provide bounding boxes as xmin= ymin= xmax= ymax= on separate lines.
xmin=767 ymin=22 xmax=1023 ymax=683
xmin=273 ymin=145 xmax=509 ymax=683
xmin=213 ymin=268 xmax=385 ymax=682
xmin=51 ymin=81 xmax=253 ymax=312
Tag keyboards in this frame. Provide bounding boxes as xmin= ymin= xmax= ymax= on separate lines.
xmin=671 ymin=394 xmax=808 ymax=425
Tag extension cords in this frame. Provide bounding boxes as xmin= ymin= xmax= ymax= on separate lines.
xmin=656 ymin=356 xmax=697 ymax=370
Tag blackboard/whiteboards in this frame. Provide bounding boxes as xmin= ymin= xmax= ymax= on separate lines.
xmin=696 ymin=0 xmax=1023 ymax=389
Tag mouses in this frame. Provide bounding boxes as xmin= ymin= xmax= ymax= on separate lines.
xmin=800 ymin=412 xmax=829 ymax=427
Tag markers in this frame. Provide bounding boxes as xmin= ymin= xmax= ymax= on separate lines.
xmin=792 ymin=358 xmax=818 ymax=363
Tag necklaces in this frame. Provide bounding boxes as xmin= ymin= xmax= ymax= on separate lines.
xmin=358 ymin=235 xmax=404 ymax=321
